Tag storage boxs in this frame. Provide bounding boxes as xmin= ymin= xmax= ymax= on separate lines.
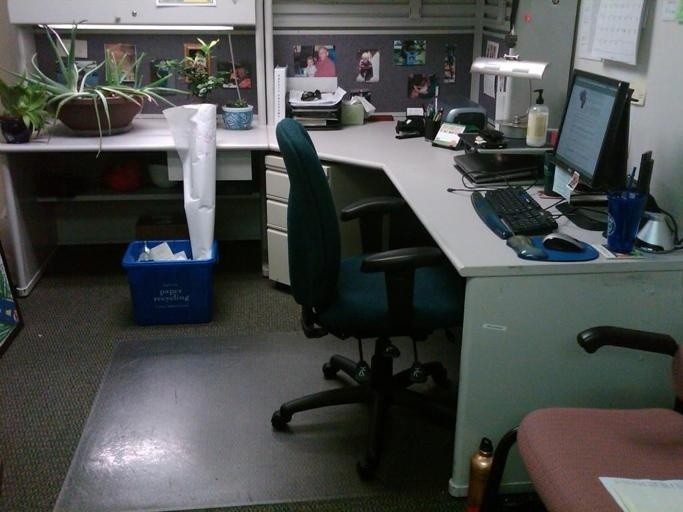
xmin=120 ymin=238 xmax=219 ymax=326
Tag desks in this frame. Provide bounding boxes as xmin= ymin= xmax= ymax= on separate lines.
xmin=0 ymin=103 xmax=681 ymax=498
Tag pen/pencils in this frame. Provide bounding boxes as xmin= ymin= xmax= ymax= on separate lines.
xmin=625 ymin=167 xmax=636 ymax=199
xmin=424 ymin=86 xmax=443 ymax=122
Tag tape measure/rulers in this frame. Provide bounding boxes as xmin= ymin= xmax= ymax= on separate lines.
xmin=637 ymin=151 xmax=654 ymax=197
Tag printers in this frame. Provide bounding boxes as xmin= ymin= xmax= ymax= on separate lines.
xmin=425 ymin=92 xmax=487 ymax=133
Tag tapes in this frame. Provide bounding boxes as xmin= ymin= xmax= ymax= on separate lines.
xmin=406 ymin=119 xmax=412 ymax=124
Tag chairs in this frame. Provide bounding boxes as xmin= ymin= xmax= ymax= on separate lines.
xmin=476 ymin=316 xmax=682 ymax=512
xmin=269 ymin=118 xmax=456 ymax=486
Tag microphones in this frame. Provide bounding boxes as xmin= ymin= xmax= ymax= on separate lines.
xmin=448 ymin=188 xmax=489 ymax=192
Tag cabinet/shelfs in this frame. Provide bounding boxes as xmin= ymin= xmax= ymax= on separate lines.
xmin=263 ymin=154 xmax=381 ymax=286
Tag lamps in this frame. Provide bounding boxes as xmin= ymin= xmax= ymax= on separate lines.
xmin=464 ymin=47 xmax=552 ymax=130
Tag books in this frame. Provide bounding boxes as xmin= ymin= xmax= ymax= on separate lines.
xmin=274 ymin=64 xmax=342 ymax=131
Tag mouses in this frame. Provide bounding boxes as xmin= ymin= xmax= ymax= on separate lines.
xmin=542 ymin=233 xmax=586 ymax=253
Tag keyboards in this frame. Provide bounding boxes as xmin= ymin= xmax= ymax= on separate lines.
xmin=471 ymin=185 xmax=558 ymax=240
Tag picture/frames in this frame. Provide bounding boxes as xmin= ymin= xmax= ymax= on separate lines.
xmin=183 ymin=40 xmax=211 ymax=85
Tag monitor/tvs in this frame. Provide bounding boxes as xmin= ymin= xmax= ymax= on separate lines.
xmin=552 ymin=69 xmax=630 ymax=231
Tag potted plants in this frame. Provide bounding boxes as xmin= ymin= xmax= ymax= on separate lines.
xmin=0 ymin=19 xmax=192 ymax=159
xmin=222 ymin=98 xmax=255 ymax=131
xmin=0 ymin=68 xmax=53 ymax=144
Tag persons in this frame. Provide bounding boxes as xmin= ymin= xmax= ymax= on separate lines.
xmin=303 ymin=48 xmax=336 ymax=77
xmin=358 ymin=52 xmax=373 ymax=81
xmin=399 ymin=41 xmax=419 ymax=65
xmin=409 ymin=74 xmax=439 ymax=99
xmin=193 ymin=51 xmax=206 ymax=67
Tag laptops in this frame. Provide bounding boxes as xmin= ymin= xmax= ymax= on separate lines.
xmin=454 ymin=149 xmax=544 ymax=184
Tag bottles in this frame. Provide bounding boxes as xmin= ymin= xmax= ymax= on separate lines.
xmin=463 ymin=437 xmax=493 ymax=512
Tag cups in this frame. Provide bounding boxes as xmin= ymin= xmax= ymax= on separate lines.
xmin=424 ymin=117 xmax=440 ymax=142
xmin=605 ymin=191 xmax=647 ymax=254
